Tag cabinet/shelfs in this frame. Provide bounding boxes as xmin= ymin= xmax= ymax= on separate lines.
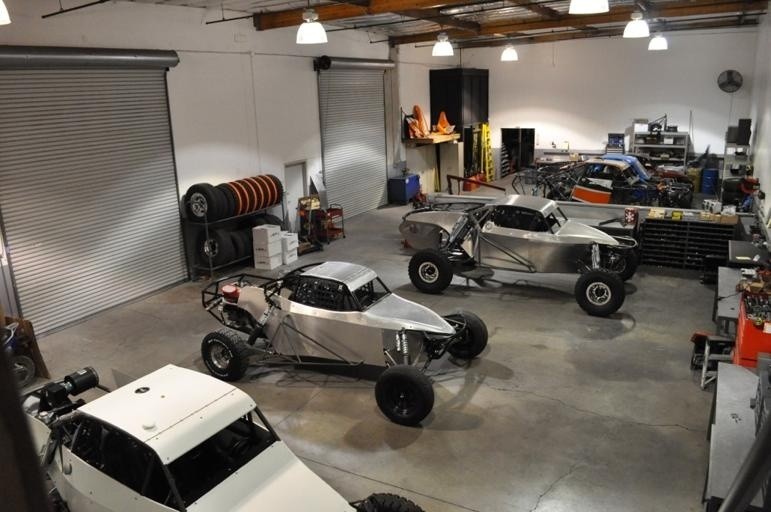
xmin=180 ymin=192 xmax=292 ymax=280
xmin=633 ymin=132 xmax=690 ymax=180
xmin=430 ymin=69 xmax=489 ymax=189
xmin=317 ymin=203 xmax=346 ymax=244
xmin=721 ymin=133 xmax=750 ymax=202
xmin=643 ymin=212 xmax=737 ymax=278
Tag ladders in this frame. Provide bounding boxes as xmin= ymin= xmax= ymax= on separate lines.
xmin=480 ymin=121 xmax=495 ymax=183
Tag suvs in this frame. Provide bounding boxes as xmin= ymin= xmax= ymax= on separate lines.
xmin=399 ymin=194 xmax=640 ymax=317
xmin=201 ymin=261 xmax=488 ymax=424
xmin=511 ymin=154 xmax=694 ymax=207
xmin=19 ymin=363 xmax=426 ymax=512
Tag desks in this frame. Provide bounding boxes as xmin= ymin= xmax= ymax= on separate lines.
xmin=706 ymin=359 xmax=771 ymax=512
xmin=712 ymin=266 xmax=743 ymax=336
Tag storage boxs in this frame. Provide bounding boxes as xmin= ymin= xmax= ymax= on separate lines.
xmin=251 ymin=222 xmax=298 ymax=269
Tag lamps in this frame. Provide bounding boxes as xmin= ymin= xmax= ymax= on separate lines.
xmin=499 ymin=36 xmax=519 ymax=63
xmin=431 ymin=24 xmax=454 ymax=57
xmin=295 ymin=1 xmax=330 ymax=46
xmin=623 ymin=3 xmax=651 ymax=39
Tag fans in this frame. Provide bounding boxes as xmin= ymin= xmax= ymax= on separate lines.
xmin=718 ymin=69 xmax=743 ymax=93
